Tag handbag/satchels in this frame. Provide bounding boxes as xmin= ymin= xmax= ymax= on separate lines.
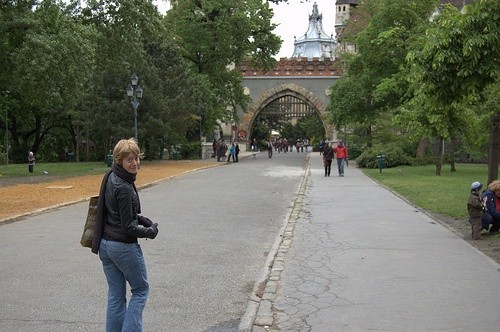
xmin=323 ymin=155 xmax=329 ymax=160
xmin=80 ymin=197 xmax=101 ymax=247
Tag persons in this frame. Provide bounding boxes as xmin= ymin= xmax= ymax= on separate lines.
xmin=250 ymin=137 xmax=258 ymax=159
xmin=211 ymin=138 xmax=228 ymax=162
xmin=467 ymin=180 xmax=500 ymax=240
xmin=267 ymin=137 xmax=310 ymax=159
xmin=227 ymin=142 xmax=240 ymax=163
xmin=98 ymin=138 xmax=158 ymax=332
xmin=320 ymin=139 xmax=349 ymax=177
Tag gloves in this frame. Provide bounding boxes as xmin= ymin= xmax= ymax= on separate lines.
xmin=137 ymin=216 xmax=153 ymax=227
xmin=142 ymin=223 xmax=158 ymax=240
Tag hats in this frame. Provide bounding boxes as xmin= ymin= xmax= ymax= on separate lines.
xmin=471 ymin=182 xmax=483 ymax=191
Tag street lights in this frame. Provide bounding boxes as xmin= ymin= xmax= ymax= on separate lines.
xmin=125 ymin=73 xmax=143 ymax=139
